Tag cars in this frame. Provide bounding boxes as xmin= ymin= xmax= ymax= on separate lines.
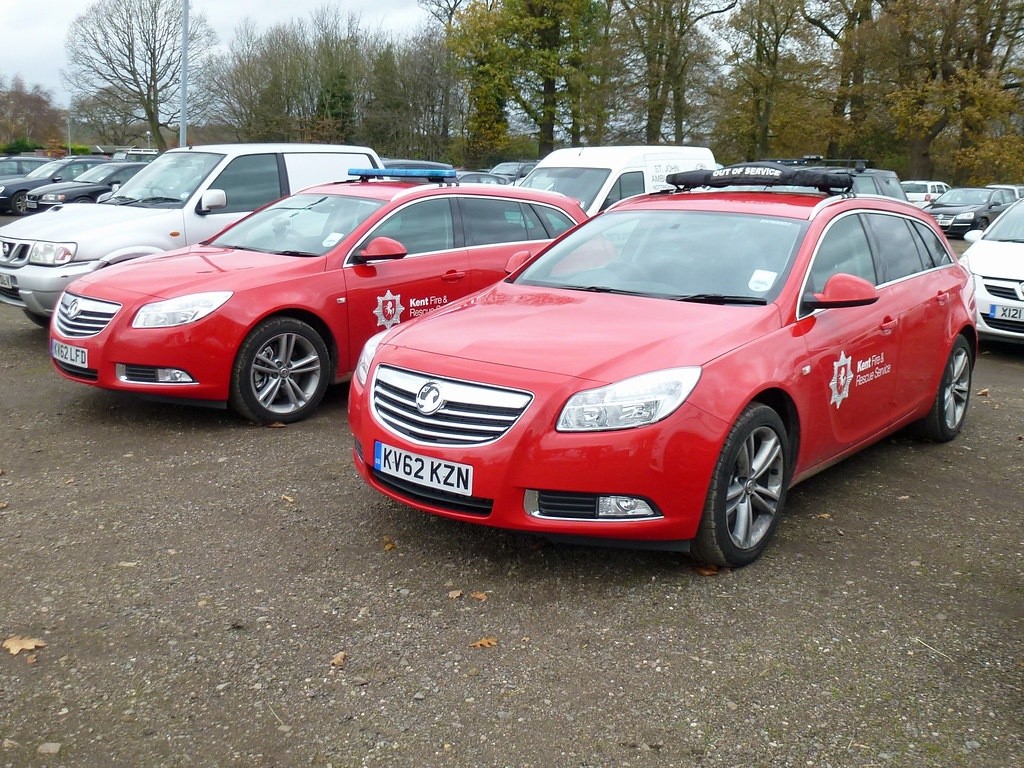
xmin=348 ymin=167 xmax=983 ymax=570
xmin=722 ymin=155 xmax=911 ymax=206
xmin=985 ymin=185 xmax=1024 ymax=200
xmin=380 ymin=156 xmax=455 ymax=184
xmin=0 ymin=153 xmax=123 ymax=215
xmin=509 ymin=176 xmax=554 ymax=192
xmin=48 ymin=160 xmax=614 ymax=428
xmin=898 ymin=179 xmax=952 ymax=212
xmin=0 ymin=157 xmax=50 ymax=177
xmin=951 ymin=194 xmax=1024 ymax=347
xmin=487 ymin=162 xmax=538 ymax=183
xmin=917 ymin=186 xmax=1020 ymax=240
xmin=110 ymin=147 xmax=160 ymax=163
xmin=23 ymin=160 xmax=153 ymax=212
xmin=456 ymin=170 xmax=512 ymax=188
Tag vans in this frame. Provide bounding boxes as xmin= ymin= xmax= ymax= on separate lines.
xmin=501 ymin=144 xmax=719 ymax=229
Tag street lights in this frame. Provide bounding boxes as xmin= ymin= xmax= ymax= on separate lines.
xmin=146 ymin=131 xmax=151 ymax=147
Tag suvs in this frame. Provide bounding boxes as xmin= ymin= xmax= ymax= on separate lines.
xmin=0 ymin=143 xmax=387 ymax=332
